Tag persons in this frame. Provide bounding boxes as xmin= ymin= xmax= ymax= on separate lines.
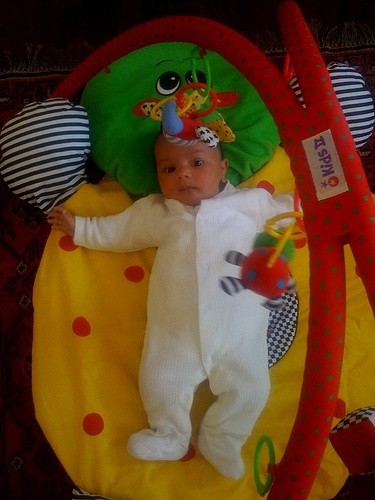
xmin=46 ymin=125 xmax=303 ymax=480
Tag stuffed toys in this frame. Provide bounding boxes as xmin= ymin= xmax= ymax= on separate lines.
xmin=219 ymin=212 xmax=307 ymax=310
